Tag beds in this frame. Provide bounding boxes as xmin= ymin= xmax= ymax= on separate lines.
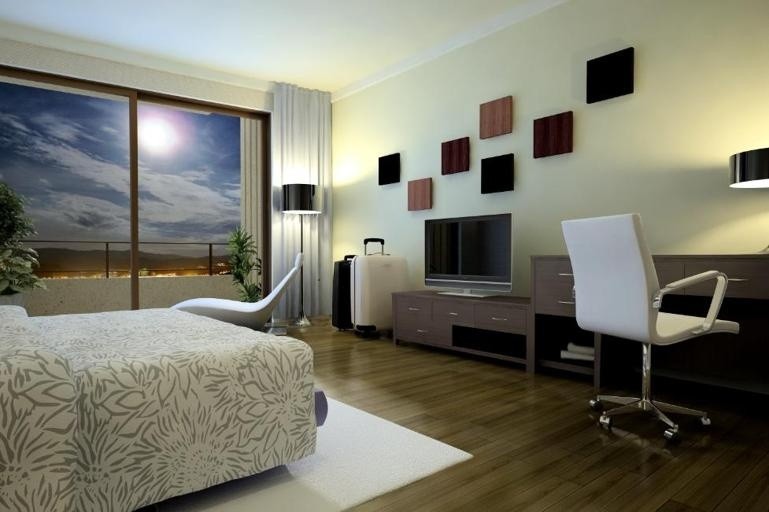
xmin=1 ymin=304 xmax=328 ymax=512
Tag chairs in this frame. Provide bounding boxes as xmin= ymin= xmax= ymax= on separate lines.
xmin=561 ymin=214 xmax=742 ymax=445
xmin=167 ymin=252 xmax=306 ymax=330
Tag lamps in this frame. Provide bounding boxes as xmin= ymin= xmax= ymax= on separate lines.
xmin=280 ymin=183 xmax=323 ymax=326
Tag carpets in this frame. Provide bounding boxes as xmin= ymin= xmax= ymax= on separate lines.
xmin=151 ymin=395 xmax=474 ymax=512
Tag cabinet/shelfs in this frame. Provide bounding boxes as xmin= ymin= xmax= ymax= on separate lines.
xmin=530 ymin=254 xmax=769 ymax=396
xmin=392 ymin=293 xmax=530 ymax=374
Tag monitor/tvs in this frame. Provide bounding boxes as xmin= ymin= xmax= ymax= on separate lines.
xmin=424 ymin=211 xmax=514 ymax=298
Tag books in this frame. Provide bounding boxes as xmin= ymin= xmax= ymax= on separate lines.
xmin=567 ymin=341 xmax=595 ymax=355
xmin=560 ymin=350 xmax=595 ymax=362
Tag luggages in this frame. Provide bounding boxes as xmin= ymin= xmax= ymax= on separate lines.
xmin=332 ymin=260 xmax=353 ymax=329
xmin=350 ymin=252 xmax=410 ymax=333
